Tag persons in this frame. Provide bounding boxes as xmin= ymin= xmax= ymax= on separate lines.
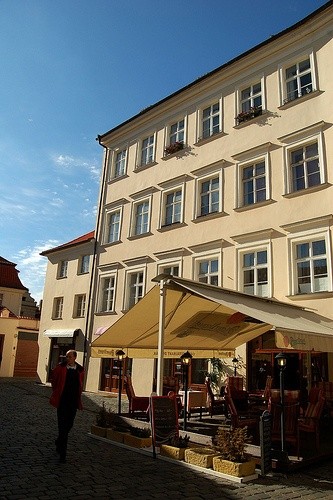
xmin=49 ymin=351 xmax=85 ymax=463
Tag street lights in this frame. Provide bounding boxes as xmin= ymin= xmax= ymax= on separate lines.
xmin=231 ymin=357 xmax=245 ymax=379
xmin=275 ymin=351 xmax=292 ymax=470
xmin=116 ymin=350 xmax=127 ymax=415
xmin=179 ymin=350 xmax=195 ymax=432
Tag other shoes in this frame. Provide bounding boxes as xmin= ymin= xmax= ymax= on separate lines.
xmin=55 ymin=438 xmax=68 ymax=464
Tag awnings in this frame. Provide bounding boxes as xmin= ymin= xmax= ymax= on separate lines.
xmin=89 ymin=274 xmax=333 ymax=396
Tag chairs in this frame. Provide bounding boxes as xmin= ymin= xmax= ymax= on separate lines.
xmin=124 ymin=374 xmax=333 ymax=460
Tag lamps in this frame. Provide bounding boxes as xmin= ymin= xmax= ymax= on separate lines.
xmin=180 ymin=351 xmax=193 ymax=365
xmin=274 ymin=350 xmax=287 ymax=366
xmin=232 ymin=358 xmax=239 ymax=367
xmin=116 ymin=350 xmax=125 ymax=361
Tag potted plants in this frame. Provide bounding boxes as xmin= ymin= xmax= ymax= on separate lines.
xmin=125 ymin=427 xmax=152 ymax=449
xmin=91 ymin=401 xmax=131 ymax=443
xmin=160 ymin=434 xmax=190 ymax=460
xmin=213 ymin=419 xmax=255 ymax=477
xmin=184 ymin=448 xmax=220 ymax=468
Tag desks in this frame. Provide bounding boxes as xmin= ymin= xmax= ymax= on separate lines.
xmin=178 ymin=391 xmax=206 ymax=421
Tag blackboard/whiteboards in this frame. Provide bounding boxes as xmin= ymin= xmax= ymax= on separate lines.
xmin=149 ymin=395 xmax=179 ymax=445
